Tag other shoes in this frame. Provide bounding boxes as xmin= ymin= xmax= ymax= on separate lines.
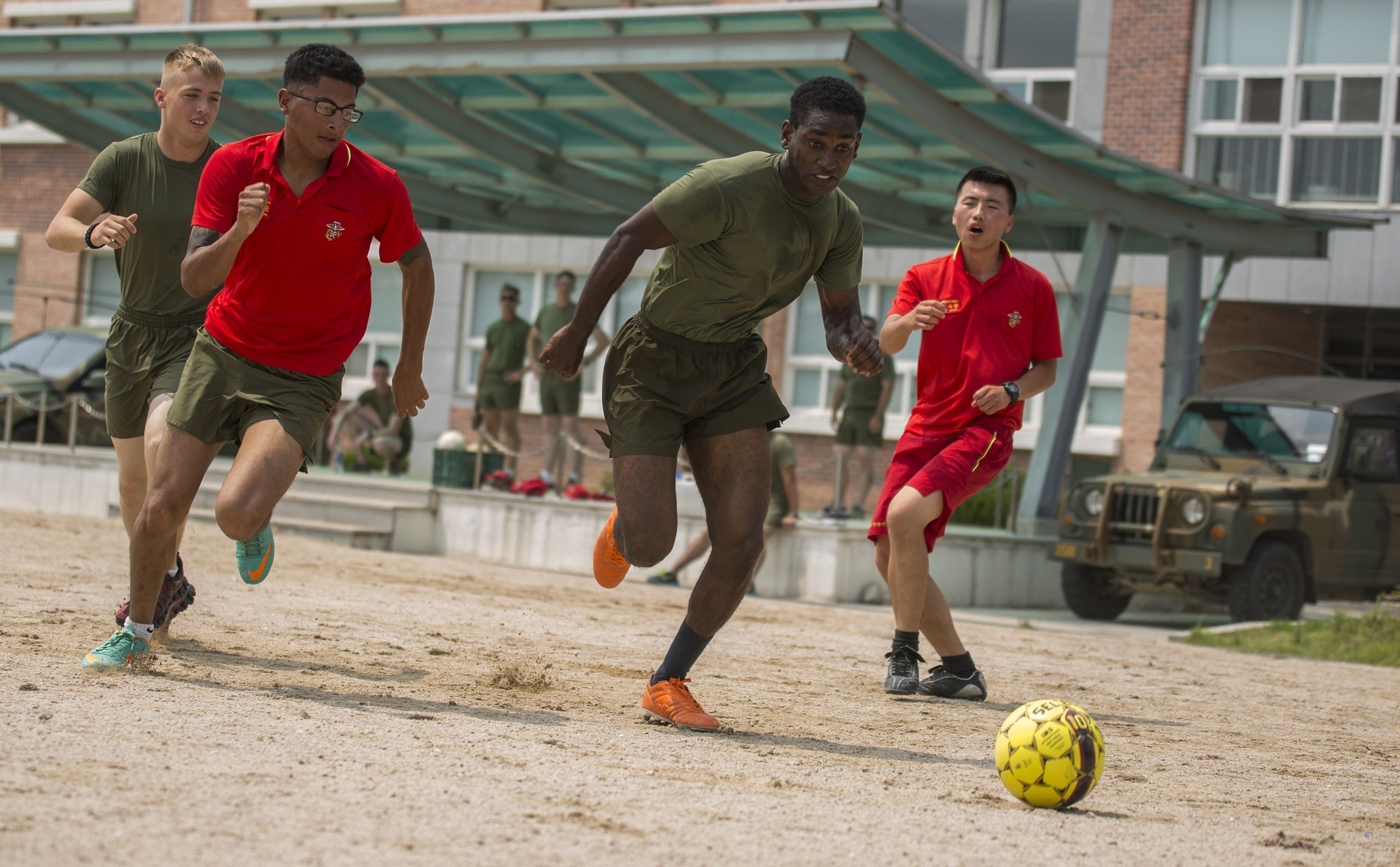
xmin=828 ymin=504 xmax=865 ymax=518
xmin=648 ymin=570 xmax=680 ymax=585
xmin=744 ymin=583 xmax=757 ymax=594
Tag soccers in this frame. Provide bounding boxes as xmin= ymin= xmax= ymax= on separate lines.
xmin=994 ymin=699 xmax=1105 ymax=808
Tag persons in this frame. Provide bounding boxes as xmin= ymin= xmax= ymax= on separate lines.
xmin=475 ymin=285 xmax=531 ymax=479
xmin=45 ymin=44 xmax=225 ymax=632
xmin=539 ymin=77 xmax=884 ymax=731
xmin=823 ymin=316 xmax=896 ymax=519
xmin=647 ymin=432 xmax=799 ymax=596
xmin=83 ymin=43 xmax=435 ymax=673
xmin=328 ymin=359 xmax=413 ymax=474
xmin=867 ymin=166 xmax=1063 ymax=702
xmin=526 ymin=272 xmax=611 ymax=491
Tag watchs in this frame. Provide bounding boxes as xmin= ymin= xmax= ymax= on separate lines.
xmin=1002 ymin=381 xmax=1019 ymax=404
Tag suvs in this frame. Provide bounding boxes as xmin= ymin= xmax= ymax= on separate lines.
xmin=1046 ymin=373 xmax=1400 ymax=624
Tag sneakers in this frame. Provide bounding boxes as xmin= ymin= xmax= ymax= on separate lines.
xmin=592 ymin=503 xmax=630 ymax=588
xmin=642 ymin=672 xmax=719 ymax=731
xmin=884 ymin=646 xmax=926 ymax=694
xmin=238 ymin=523 xmax=275 ymax=585
xmin=116 ymin=553 xmax=190 ymax=628
xmin=82 ymin=625 xmax=153 ymax=670
xmin=162 ymin=585 xmax=196 ymax=627
xmin=916 ymin=665 xmax=988 ymax=700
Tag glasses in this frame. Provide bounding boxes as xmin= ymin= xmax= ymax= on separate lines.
xmin=501 ymin=295 xmax=517 ymax=302
xmin=286 ymin=90 xmax=365 ymax=124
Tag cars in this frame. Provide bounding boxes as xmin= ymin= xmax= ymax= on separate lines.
xmin=0 ymin=325 xmax=114 ymax=446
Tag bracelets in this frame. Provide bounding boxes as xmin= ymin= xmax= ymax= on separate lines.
xmin=875 ymin=412 xmax=883 ymax=419
xmin=84 ymin=223 xmax=106 ymax=249
xmin=582 ymin=357 xmax=587 ymax=365
xmin=788 ymin=511 xmax=797 ymax=517
